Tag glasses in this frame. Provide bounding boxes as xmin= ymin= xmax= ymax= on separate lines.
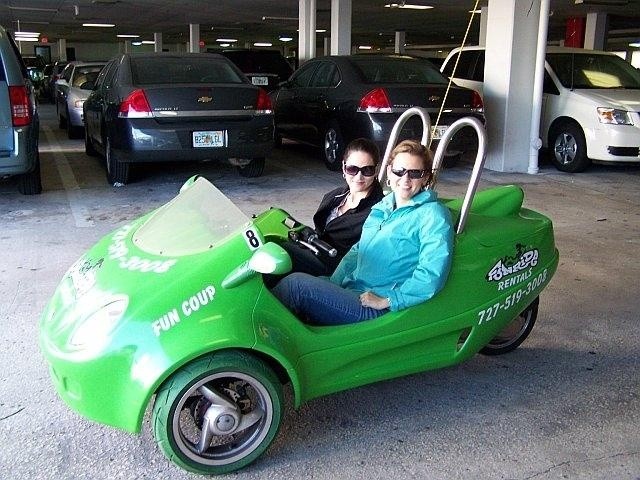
xmin=344 ymin=161 xmax=378 ymax=177
xmin=391 ymin=168 xmax=431 ymax=179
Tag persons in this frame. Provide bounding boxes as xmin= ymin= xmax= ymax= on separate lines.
xmin=266 ymin=137 xmax=384 ymax=275
xmin=269 ymin=138 xmax=454 ymax=325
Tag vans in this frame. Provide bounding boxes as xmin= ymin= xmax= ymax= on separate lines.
xmin=1 ymin=26 xmax=47 ymax=197
